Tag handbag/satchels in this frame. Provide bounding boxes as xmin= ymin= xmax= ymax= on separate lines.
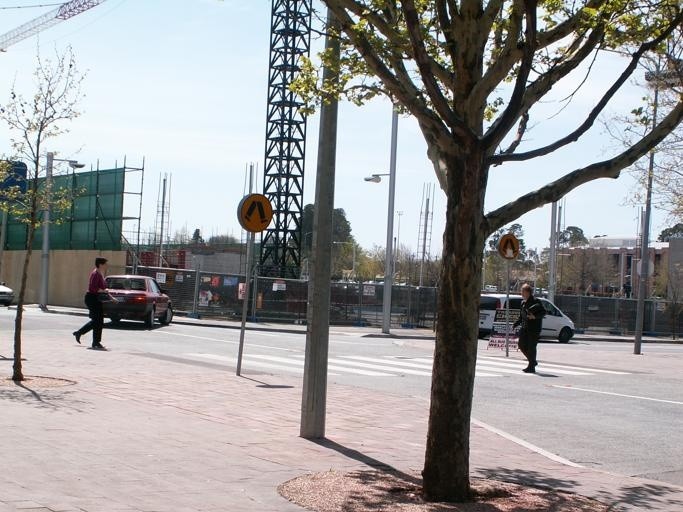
xmin=528 ymin=303 xmax=540 ymax=314
xmin=99 ymin=293 xmax=112 ymax=302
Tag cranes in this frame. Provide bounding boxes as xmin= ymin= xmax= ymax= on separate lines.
xmin=0 ymin=0 xmax=105 ymax=51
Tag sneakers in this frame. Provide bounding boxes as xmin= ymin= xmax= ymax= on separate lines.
xmin=73 ymin=331 xmax=81 ymax=343
xmin=92 ymin=342 xmax=104 ymax=348
xmin=523 ymin=361 xmax=537 ymax=373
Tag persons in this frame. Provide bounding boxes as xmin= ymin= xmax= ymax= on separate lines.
xmin=73 ymin=257 xmax=118 ymax=350
xmin=206 ymin=287 xmax=213 ymax=305
xmin=512 ymin=284 xmax=546 ymax=373
xmin=585 ymin=285 xmax=593 ymax=296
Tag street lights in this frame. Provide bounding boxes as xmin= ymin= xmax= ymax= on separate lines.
xmin=333 ymin=241 xmax=355 ymax=283
xmin=40 ymin=152 xmax=85 ymax=305
xmin=363 ymin=173 xmax=396 ymax=334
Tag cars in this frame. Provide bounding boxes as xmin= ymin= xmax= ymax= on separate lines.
xmin=532 ymin=288 xmax=548 ymax=295
xmin=0 ymin=282 xmax=14 ymax=306
xmin=484 ymin=285 xmax=498 ymax=291
xmin=103 ymin=274 xmax=173 ymax=327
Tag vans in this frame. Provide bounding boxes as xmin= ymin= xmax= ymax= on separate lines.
xmin=478 ymin=294 xmax=575 ymax=343
